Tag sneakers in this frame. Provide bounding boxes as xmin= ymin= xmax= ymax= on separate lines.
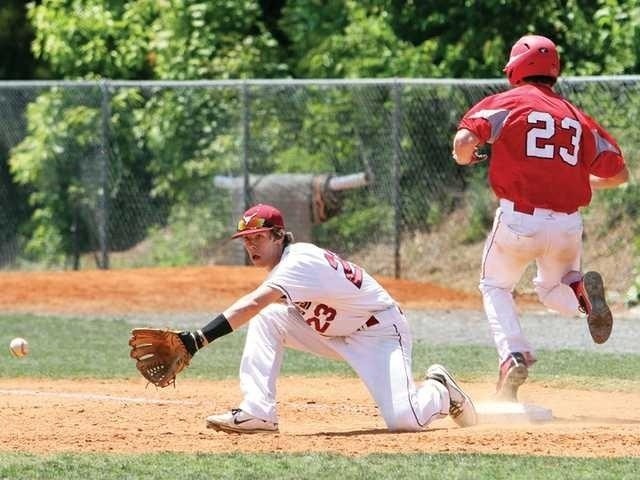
xmin=425 ymin=364 xmax=480 ymax=427
xmin=577 ymin=271 xmax=613 ymax=345
xmin=491 ymin=353 xmax=528 ymax=406
xmin=205 ymin=409 xmax=281 ymax=436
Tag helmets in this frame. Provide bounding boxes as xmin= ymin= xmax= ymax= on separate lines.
xmin=504 ymin=34 xmax=560 ymax=85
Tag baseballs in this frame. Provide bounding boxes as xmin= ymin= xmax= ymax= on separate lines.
xmin=9 ymin=338 xmax=29 ymax=358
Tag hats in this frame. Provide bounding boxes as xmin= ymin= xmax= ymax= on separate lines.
xmin=230 ymin=203 xmax=286 ymax=238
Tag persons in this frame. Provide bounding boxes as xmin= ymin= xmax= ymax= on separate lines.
xmin=449 ymin=34 xmax=633 ymax=403
xmin=127 ymin=197 xmax=482 ymax=436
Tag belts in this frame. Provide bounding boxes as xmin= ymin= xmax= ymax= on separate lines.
xmin=358 ymin=315 xmax=379 ymax=331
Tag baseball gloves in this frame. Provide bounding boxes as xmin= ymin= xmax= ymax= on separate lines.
xmin=129 ymin=328 xmax=204 ymax=387
